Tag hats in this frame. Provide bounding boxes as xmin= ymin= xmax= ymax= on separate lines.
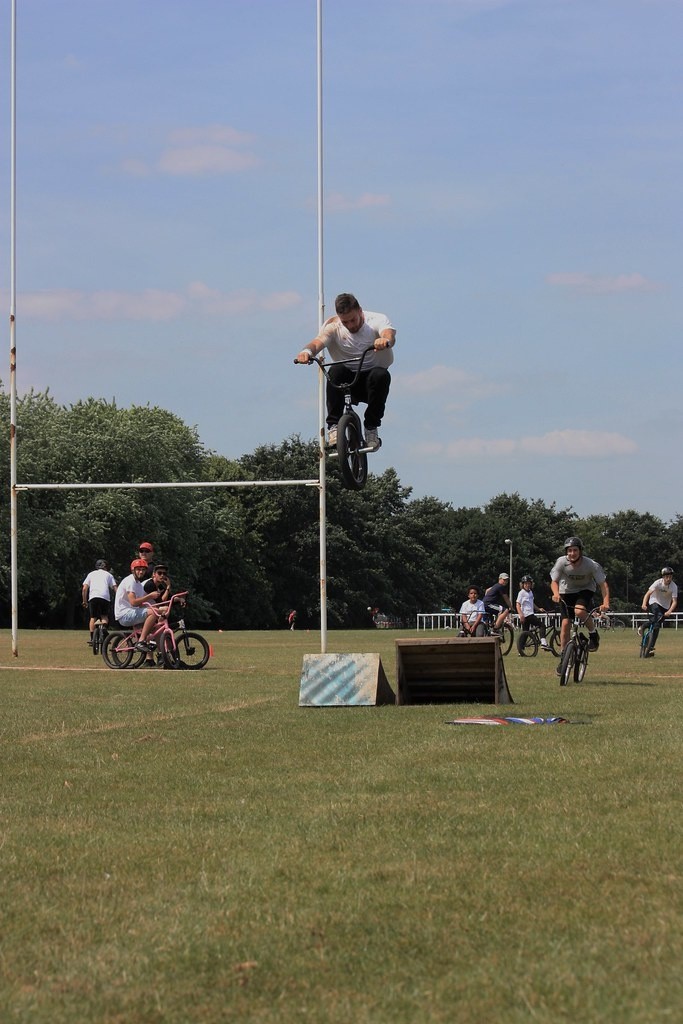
xmin=139 ymin=542 xmax=153 ymax=551
xmin=154 ymin=564 xmax=169 ymax=572
xmin=499 ymin=573 xmax=509 ymax=579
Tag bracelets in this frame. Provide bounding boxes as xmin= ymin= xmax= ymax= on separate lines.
xmin=300 ymin=348 xmax=313 ymax=359
xmin=387 ymin=339 xmax=390 ymax=347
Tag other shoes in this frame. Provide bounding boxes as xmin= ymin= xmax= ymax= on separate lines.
xmin=491 ymin=629 xmax=504 ymax=636
xmin=541 ymin=643 xmax=553 ymax=651
xmin=638 ymin=627 xmax=643 ymax=637
xmin=146 ymin=659 xmax=156 ymax=666
xmin=518 ymin=653 xmax=525 ymax=655
xmin=649 ymin=650 xmax=654 ymax=654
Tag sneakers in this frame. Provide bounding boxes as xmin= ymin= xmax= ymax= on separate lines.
xmin=364 ymin=427 xmax=379 ymax=452
xmin=135 ymin=642 xmax=151 ymax=652
xmin=156 ymin=652 xmax=164 ymax=665
xmin=328 ymin=424 xmax=337 ymax=446
xmin=588 ymin=629 xmax=600 ymax=651
xmin=555 ymin=652 xmax=567 ymax=675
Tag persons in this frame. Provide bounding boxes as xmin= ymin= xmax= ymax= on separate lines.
xmin=297 ymin=293 xmax=396 ymax=453
xmin=638 ymin=567 xmax=677 ymax=653
xmin=516 ymin=575 xmax=554 ymax=657
xmin=482 ymin=573 xmax=514 ymax=636
xmin=549 ymin=537 xmax=610 ymax=676
xmin=82 ymin=560 xmax=118 ymax=642
xmin=114 ymin=542 xmax=185 ymax=668
xmin=459 ymin=585 xmax=485 ymax=637
xmin=289 ymin=611 xmax=296 ymax=631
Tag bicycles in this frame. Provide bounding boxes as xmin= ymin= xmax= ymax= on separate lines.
xmin=102 ymin=591 xmax=189 ymax=669
xmin=293 ymin=341 xmax=391 ymax=492
xmin=635 ymin=604 xmax=669 ymax=658
xmin=86 ymin=596 xmax=115 ymax=655
xmin=449 ymin=606 xmax=516 ymax=656
xmin=110 ymin=601 xmax=210 ymax=670
xmin=517 ymin=609 xmax=561 ymax=658
xmin=548 ymin=596 xmax=613 ymax=686
xmin=594 ymin=615 xmax=626 ymax=634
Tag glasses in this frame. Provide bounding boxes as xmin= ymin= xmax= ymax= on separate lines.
xmin=139 ymin=548 xmax=151 ymax=553
xmin=156 ymin=571 xmax=167 ymax=576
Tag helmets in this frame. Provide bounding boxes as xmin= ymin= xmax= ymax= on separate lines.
xmin=563 ymin=537 xmax=585 ymax=549
xmin=661 ymin=567 xmax=674 ymax=576
xmin=521 ymin=575 xmax=533 ymax=584
xmin=95 ymin=559 xmax=108 ymax=569
xmin=131 ymin=559 xmax=148 ymax=571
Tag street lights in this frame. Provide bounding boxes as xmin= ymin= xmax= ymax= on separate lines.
xmin=504 ymin=539 xmax=513 ymax=610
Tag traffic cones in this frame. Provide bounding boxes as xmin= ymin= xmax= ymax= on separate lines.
xmin=207 ymin=644 xmax=214 ymax=657
